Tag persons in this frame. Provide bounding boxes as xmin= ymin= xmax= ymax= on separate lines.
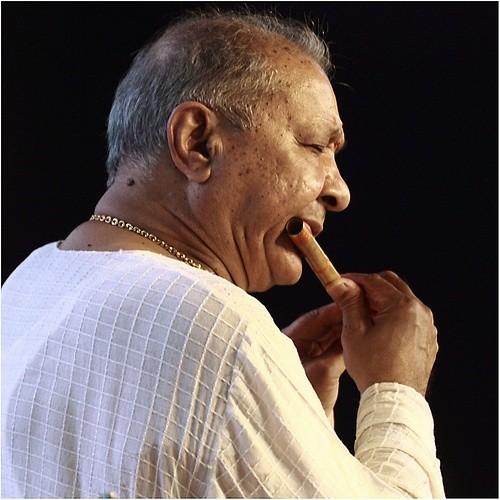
xmin=1 ymin=11 xmax=447 ymax=500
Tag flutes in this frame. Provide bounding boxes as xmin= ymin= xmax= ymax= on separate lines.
xmin=286 ymin=218 xmax=340 ymax=287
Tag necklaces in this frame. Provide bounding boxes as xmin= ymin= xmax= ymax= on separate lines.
xmin=88 ymin=213 xmax=210 ymax=270
